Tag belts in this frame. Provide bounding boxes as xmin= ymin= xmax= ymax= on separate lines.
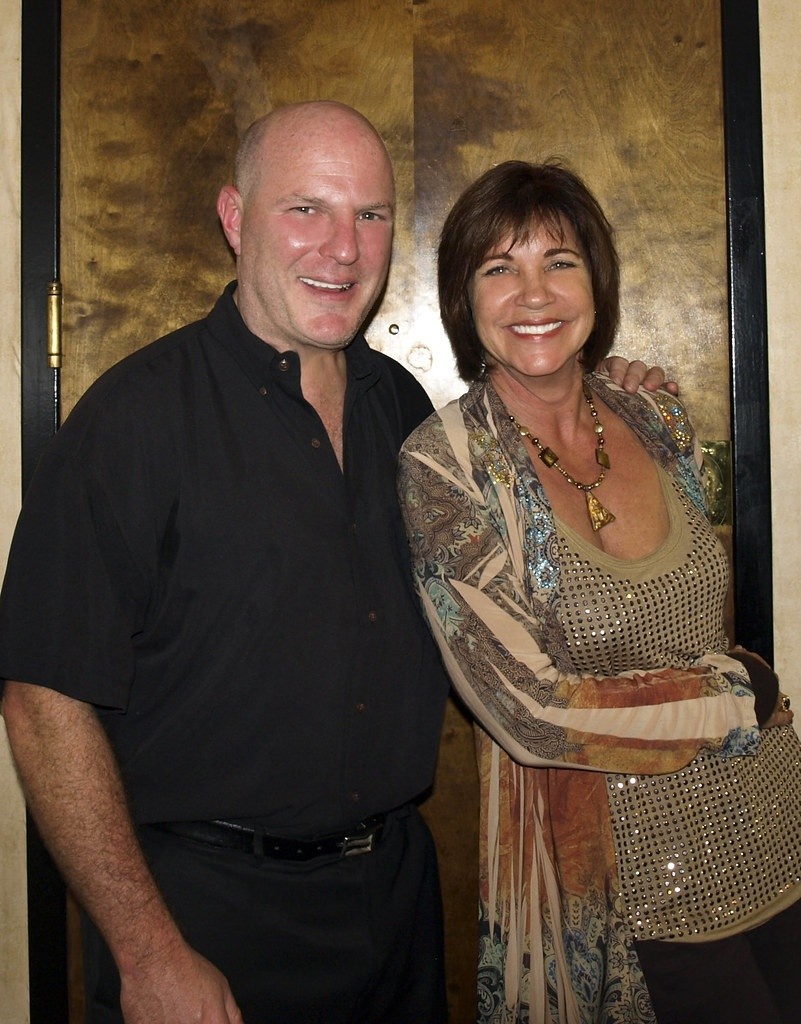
xmin=143 ymin=810 xmax=391 ymax=861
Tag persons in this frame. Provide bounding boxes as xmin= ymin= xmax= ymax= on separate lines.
xmin=393 ymin=154 xmax=800 ymax=1024
xmin=0 ymin=97 xmax=683 ymax=1021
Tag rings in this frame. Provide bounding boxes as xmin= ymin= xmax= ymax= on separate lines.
xmin=781 ymin=693 xmax=791 ymax=713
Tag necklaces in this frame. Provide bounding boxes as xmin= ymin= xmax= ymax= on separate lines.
xmin=506 ymin=375 xmax=616 ymax=533
